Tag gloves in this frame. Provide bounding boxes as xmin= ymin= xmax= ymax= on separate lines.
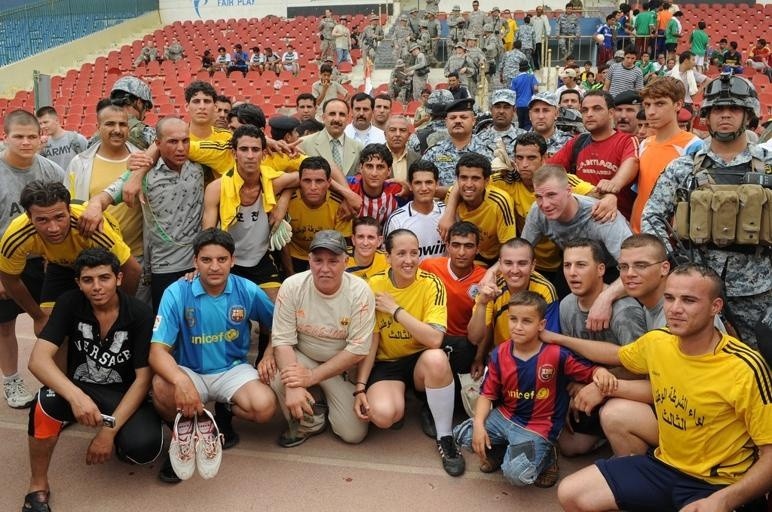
xmin=268 ymin=219 xmax=294 ymax=253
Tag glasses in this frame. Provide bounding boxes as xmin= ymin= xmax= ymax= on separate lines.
xmin=616 ymin=259 xmax=666 ymax=272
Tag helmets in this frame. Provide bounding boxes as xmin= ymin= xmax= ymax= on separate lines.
xmin=109 ymin=76 xmax=153 ymax=120
xmin=699 ymin=66 xmax=761 ymax=143
xmin=554 ymin=107 xmax=585 ymax=133
xmin=426 ymin=88 xmax=454 ymax=118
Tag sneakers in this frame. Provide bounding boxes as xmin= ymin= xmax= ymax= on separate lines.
xmin=159 ymin=456 xmax=181 ymax=484
xmin=418 ymin=402 xmax=437 ymax=438
xmin=479 ymin=456 xmax=503 ymax=473
xmin=534 ymin=462 xmax=560 ymax=489
xmin=278 ymin=421 xmax=326 ymax=448
xmin=436 ymin=435 xmax=466 ymax=477
xmin=193 ymin=408 xmax=225 ymax=481
xmin=168 ymin=410 xmax=197 ymax=481
xmin=213 ymin=401 xmax=239 ymax=450
xmin=2 ymin=375 xmax=35 ymax=409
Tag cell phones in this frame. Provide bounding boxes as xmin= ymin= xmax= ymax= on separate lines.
xmin=99 ymin=413 xmax=116 ymax=428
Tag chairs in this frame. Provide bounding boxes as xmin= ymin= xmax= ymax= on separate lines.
xmin=0 ymin=1 xmax=448 ymax=143
xmin=451 ymin=11 xmax=607 ymax=48
xmin=670 ymin=3 xmax=771 ymax=142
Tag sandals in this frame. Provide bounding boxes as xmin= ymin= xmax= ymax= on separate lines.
xmin=22 ymin=490 xmax=52 ymax=512
xmin=115 ymin=445 xmax=136 ymax=465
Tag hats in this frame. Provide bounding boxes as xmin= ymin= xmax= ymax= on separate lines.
xmin=636 ymin=109 xmax=647 ymax=121
xmin=614 ymin=90 xmax=643 ymax=106
xmin=527 ymin=90 xmax=558 ymax=107
xmin=677 ymin=108 xmax=692 ymax=123
xmin=308 ymin=229 xmax=349 ymax=256
xmin=491 ymin=88 xmax=517 ymax=106
xmin=337 ymin=3 xmax=625 ymax=80
xmin=444 ymin=98 xmax=476 ymax=112
xmin=268 ymin=114 xmax=301 ymax=131
